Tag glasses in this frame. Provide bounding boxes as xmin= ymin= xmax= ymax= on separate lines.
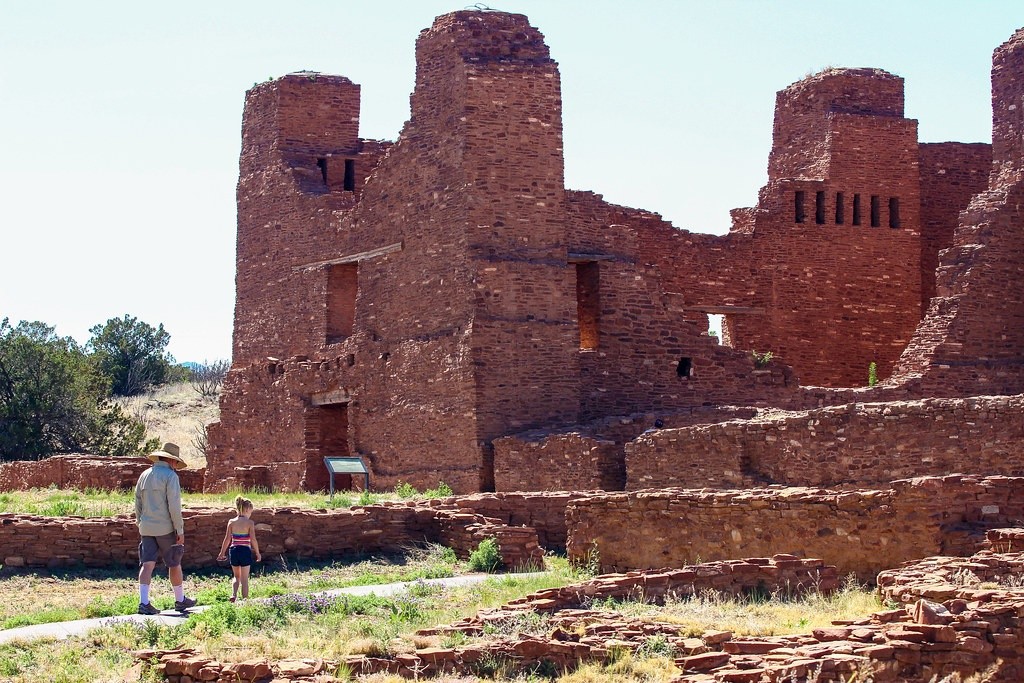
xmin=176 ymin=461 xmax=179 ymax=464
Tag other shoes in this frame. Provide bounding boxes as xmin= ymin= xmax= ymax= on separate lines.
xmin=229 ymin=596 xmax=235 ymax=602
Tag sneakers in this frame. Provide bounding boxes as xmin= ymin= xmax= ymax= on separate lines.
xmin=138 ymin=602 xmax=160 ymax=615
xmin=175 ymin=596 xmax=197 ymax=611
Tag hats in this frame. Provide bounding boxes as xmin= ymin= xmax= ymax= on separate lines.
xmin=148 ymin=442 xmax=187 ymax=470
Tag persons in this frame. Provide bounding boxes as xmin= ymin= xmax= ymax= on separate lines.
xmin=134 ymin=442 xmax=199 ymax=614
xmin=217 ymin=495 xmax=265 ymax=602
